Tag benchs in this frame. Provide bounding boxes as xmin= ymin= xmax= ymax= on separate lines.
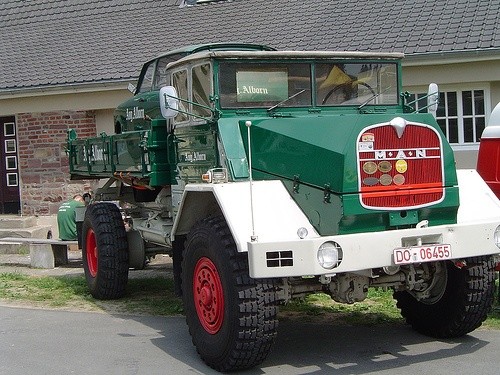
xmin=30 ymin=237 xmax=82 ymax=269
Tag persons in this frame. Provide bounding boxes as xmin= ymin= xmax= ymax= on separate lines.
xmin=57 ymin=195 xmax=85 ymax=242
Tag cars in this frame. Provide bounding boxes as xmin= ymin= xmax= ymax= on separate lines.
xmin=112 ymin=44 xmax=276 ymax=132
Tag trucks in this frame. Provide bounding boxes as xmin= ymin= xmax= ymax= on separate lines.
xmin=64 ymin=51 xmax=500 ymax=372
